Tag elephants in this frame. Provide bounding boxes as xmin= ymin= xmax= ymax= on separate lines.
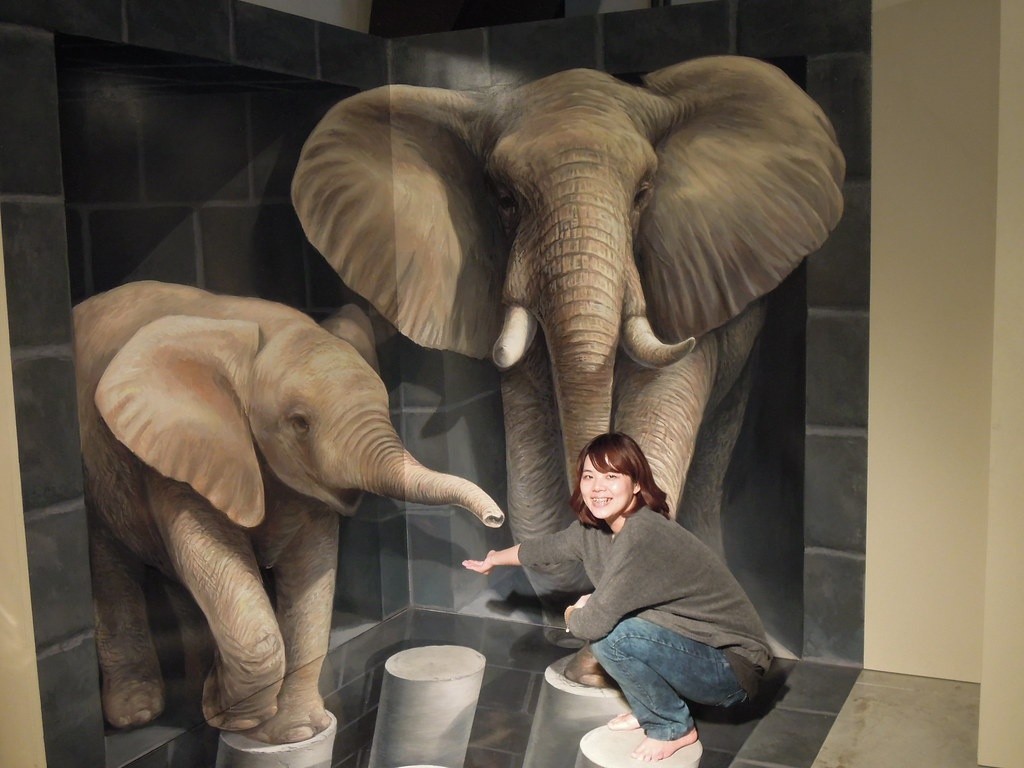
xmin=292 ymin=55 xmax=845 ymax=649
xmin=73 ymin=280 xmax=504 ymax=744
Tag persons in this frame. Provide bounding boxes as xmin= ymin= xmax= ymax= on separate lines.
xmin=461 ymin=431 xmax=773 ymax=762
